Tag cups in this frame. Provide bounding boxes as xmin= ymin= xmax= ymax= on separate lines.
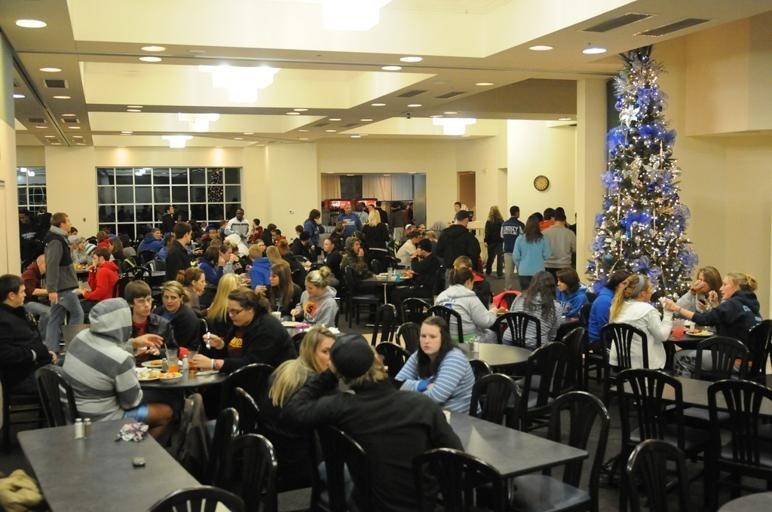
xmin=187 ymin=351 xmax=198 ymax=373
xmin=396 ymin=272 xmax=400 ymax=280
xmin=442 ymin=410 xmax=451 ymax=424
xmin=317 ymin=224 xmax=335 ymax=234
xmin=272 ymin=312 xmax=281 ymax=321
xmin=387 ymin=267 xmax=392 ymax=276
xmin=672 ymin=319 xmax=683 ymax=338
xmin=166 ymin=348 xmax=179 ymax=372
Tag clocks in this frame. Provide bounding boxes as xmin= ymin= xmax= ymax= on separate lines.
xmin=533 ymin=175 xmax=550 ymax=192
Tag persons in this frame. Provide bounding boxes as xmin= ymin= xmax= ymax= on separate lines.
xmin=193 ymin=288 xmax=297 ymax=422
xmin=282 ymin=335 xmax=463 ymax=511
xmin=394 ymin=316 xmax=482 ymax=417
xmin=16 ymin=201 xmax=584 ymax=378
xmin=1 ymin=273 xmax=69 ymax=394
xmin=585 ymin=263 xmax=764 ymax=382
xmin=254 ymin=323 xmax=339 ymax=494
xmin=63 ymin=296 xmax=173 ymax=443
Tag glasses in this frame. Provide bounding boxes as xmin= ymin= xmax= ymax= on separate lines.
xmin=227 ymin=308 xmax=246 ymax=314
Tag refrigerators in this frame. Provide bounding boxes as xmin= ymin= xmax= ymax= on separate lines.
xmin=322 ymin=198 xmax=378 ymax=226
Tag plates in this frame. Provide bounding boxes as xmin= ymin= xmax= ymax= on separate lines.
xmin=399 ymin=277 xmax=409 ymax=279
xmin=282 ymin=321 xmax=305 ymax=328
xmin=685 ymin=329 xmax=714 ymax=337
xmin=160 ymin=373 xmax=183 ymax=383
xmin=138 ymin=369 xmax=161 ymax=381
xmin=141 ymin=359 xmax=162 ymax=367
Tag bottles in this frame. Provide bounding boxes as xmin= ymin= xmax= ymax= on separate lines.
xmin=183 ymin=354 xmax=188 ymax=370
xmin=84 ymin=417 xmax=92 ymax=438
xmin=162 ymin=358 xmax=168 ymax=372
xmin=74 ymin=417 xmax=84 ymax=439
xmin=468 ymin=338 xmax=479 ymax=352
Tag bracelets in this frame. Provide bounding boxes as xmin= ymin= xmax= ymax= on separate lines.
xmin=210 ymin=358 xmax=215 ymax=370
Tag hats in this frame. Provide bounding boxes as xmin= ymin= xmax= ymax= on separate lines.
xmin=330 ymin=332 xmax=375 ymax=380
